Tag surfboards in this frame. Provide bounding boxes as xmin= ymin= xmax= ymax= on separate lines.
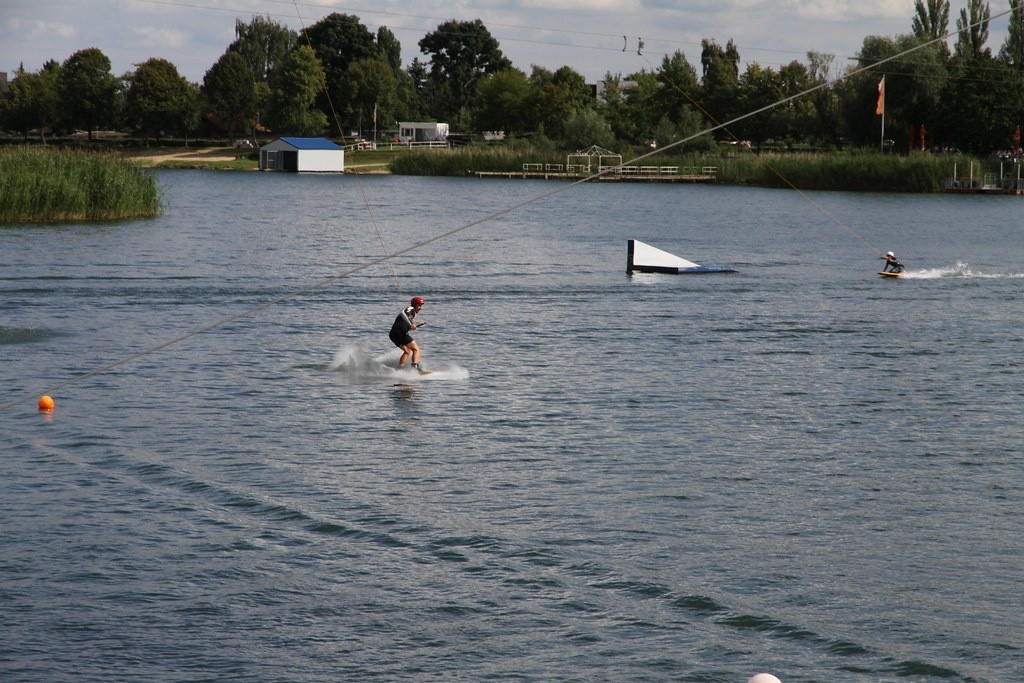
xmin=878 ymin=272 xmax=902 ymax=275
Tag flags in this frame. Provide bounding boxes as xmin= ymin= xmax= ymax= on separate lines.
xmin=876 ymin=76 xmax=884 ymax=114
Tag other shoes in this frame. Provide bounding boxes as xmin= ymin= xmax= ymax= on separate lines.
xmin=411 ymin=363 xmax=422 ymax=371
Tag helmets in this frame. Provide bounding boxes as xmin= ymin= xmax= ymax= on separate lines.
xmin=411 ymin=296 xmax=424 ymax=306
xmin=887 ymin=251 xmax=894 ymax=257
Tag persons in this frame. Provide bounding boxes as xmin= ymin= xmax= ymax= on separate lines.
xmin=389 ymin=297 xmax=425 ymax=370
xmin=883 ymin=252 xmax=904 ymax=273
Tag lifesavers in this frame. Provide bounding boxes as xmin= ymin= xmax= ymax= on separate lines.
xmin=392 ymin=139 xmax=398 ymax=143
xmin=362 ymin=142 xmax=371 ymax=151
